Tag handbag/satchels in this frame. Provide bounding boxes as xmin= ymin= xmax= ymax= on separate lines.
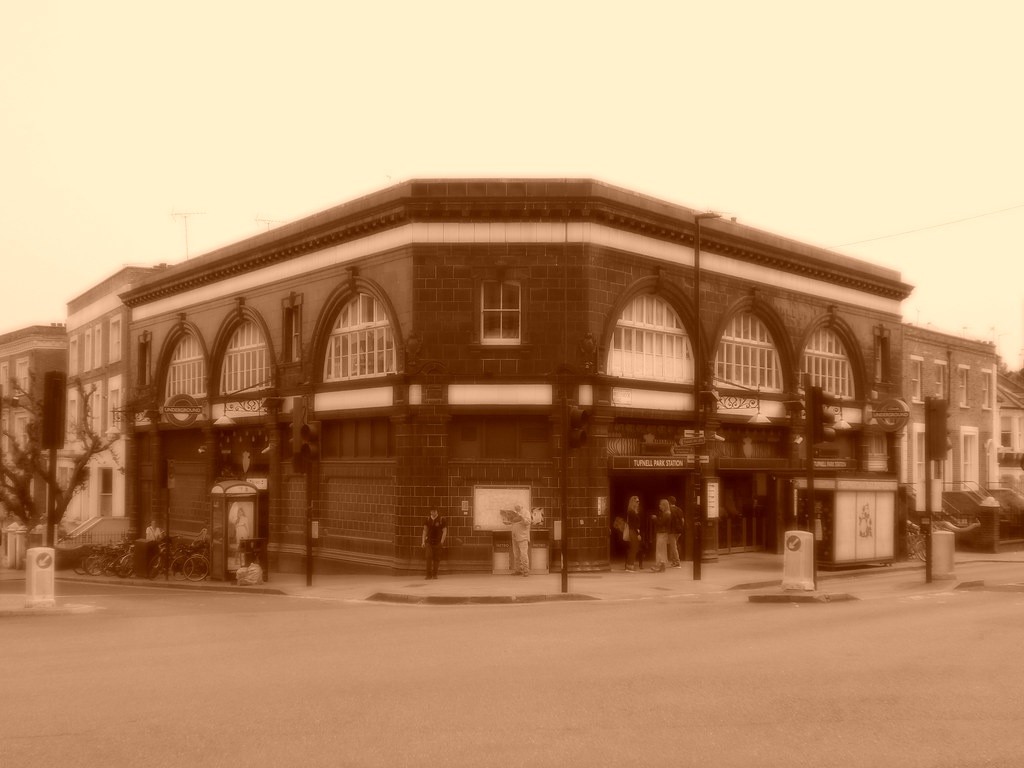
xmin=623 ymin=523 xmax=642 ymax=542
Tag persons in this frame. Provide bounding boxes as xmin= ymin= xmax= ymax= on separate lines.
xmin=421 ymin=507 xmax=448 ymax=580
xmin=511 ymin=500 xmax=531 ymax=577
xmin=624 ymin=495 xmax=643 ymax=572
xmin=145 ymin=520 xmax=161 ymax=541
xmin=234 ymin=508 xmax=249 ymax=567
xmin=650 ymin=499 xmax=672 ymax=573
xmin=666 ymin=498 xmax=685 ymax=568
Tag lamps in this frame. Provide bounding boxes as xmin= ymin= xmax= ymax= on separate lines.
xmin=716 ymin=389 xmax=772 ymax=424
xmin=198 ymin=445 xmax=207 ymax=453
xmin=214 ymin=376 xmax=274 ymax=425
xmin=823 ymin=398 xmax=852 ymax=429
xmin=260 ymin=396 xmax=282 ymax=407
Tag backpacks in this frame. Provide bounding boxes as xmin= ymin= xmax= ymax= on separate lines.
xmin=671 ymin=509 xmax=684 ymax=535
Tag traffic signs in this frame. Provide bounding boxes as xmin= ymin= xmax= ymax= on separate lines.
xmin=670 ymin=446 xmax=696 ymax=457
xmin=679 ymin=437 xmax=706 ymax=446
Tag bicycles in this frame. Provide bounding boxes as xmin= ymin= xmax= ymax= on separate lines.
xmin=69 ymin=534 xmax=211 ymax=582
xmin=907 ymin=529 xmax=927 ymax=562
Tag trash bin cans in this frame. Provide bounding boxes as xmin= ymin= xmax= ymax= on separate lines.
xmin=530 ymin=528 xmax=550 ymax=575
xmin=491 ymin=530 xmax=513 ymax=574
xmin=135 ymin=539 xmax=158 ymax=579
xmin=239 ymin=538 xmax=269 ymax=582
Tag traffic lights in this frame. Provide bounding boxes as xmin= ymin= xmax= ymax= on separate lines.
xmin=813 ymin=386 xmax=837 ymax=443
xmin=566 ymin=403 xmax=589 ymax=450
xmin=301 ymin=420 xmax=321 ymax=463
xmin=931 ymin=398 xmax=954 ymax=461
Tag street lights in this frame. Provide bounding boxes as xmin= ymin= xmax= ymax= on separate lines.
xmin=693 ymin=212 xmax=723 ymax=580
